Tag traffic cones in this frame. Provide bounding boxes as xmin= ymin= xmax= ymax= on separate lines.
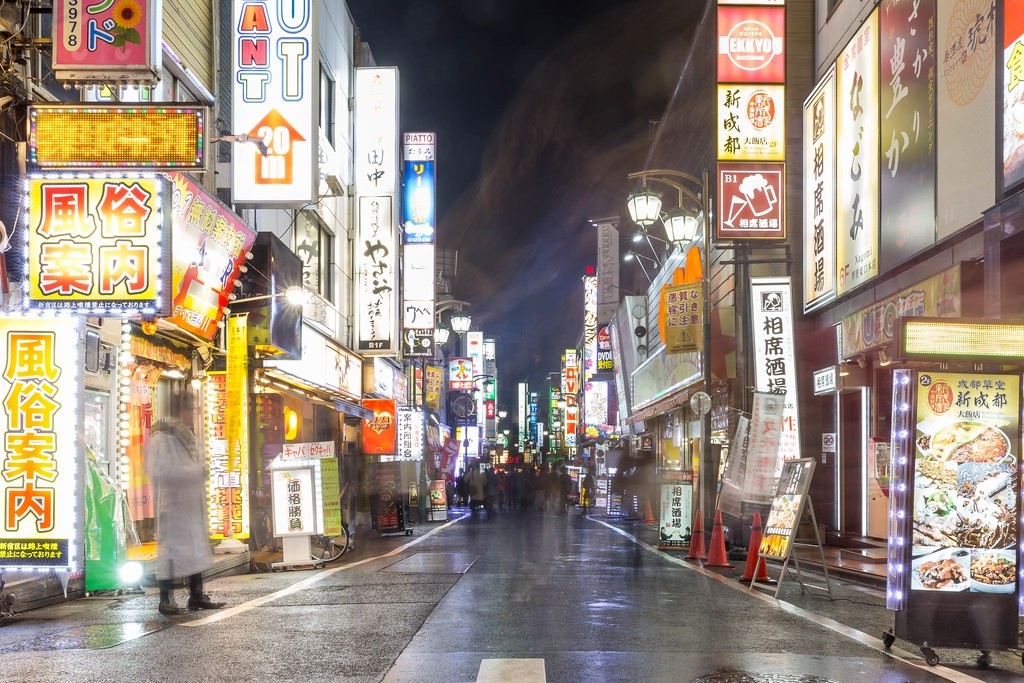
xmin=735 ymin=511 xmax=779 ymax=584
xmin=641 ymin=492 xmax=658 ymax=523
xmin=684 ymin=510 xmax=710 ymax=560
xmin=704 ymin=509 xmax=736 ymax=569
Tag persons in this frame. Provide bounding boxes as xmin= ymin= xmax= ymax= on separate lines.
xmin=146 ymin=391 xmax=226 ymax=614
xmin=456 ymin=459 xmax=595 ymax=521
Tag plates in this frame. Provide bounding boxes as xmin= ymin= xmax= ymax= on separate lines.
xmin=908 ymin=420 xmax=1016 ymax=593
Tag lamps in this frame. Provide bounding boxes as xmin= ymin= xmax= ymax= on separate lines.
xmin=229 ymin=286 xmax=303 ymax=304
xmin=632 ymin=230 xmax=668 ymax=245
xmin=607 ymin=284 xmax=635 ymax=294
xmin=624 ymin=250 xmax=654 ymax=262
xmin=210 ymin=134 xmax=269 ymax=158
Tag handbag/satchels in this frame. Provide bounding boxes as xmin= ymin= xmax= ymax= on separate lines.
xmin=468 ymin=485 xmax=476 ymax=495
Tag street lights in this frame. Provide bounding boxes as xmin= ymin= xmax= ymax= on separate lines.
xmin=627 ymin=166 xmax=714 ymax=545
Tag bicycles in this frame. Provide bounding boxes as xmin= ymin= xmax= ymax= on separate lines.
xmin=308 ymin=519 xmax=355 ymax=562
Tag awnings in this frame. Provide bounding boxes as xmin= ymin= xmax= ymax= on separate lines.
xmin=271 ymin=385 xmax=374 ymax=418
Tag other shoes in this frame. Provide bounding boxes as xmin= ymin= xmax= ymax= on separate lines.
xmin=158 ymin=602 xmax=186 ymax=614
xmin=186 ymin=594 xmax=227 ymax=611
